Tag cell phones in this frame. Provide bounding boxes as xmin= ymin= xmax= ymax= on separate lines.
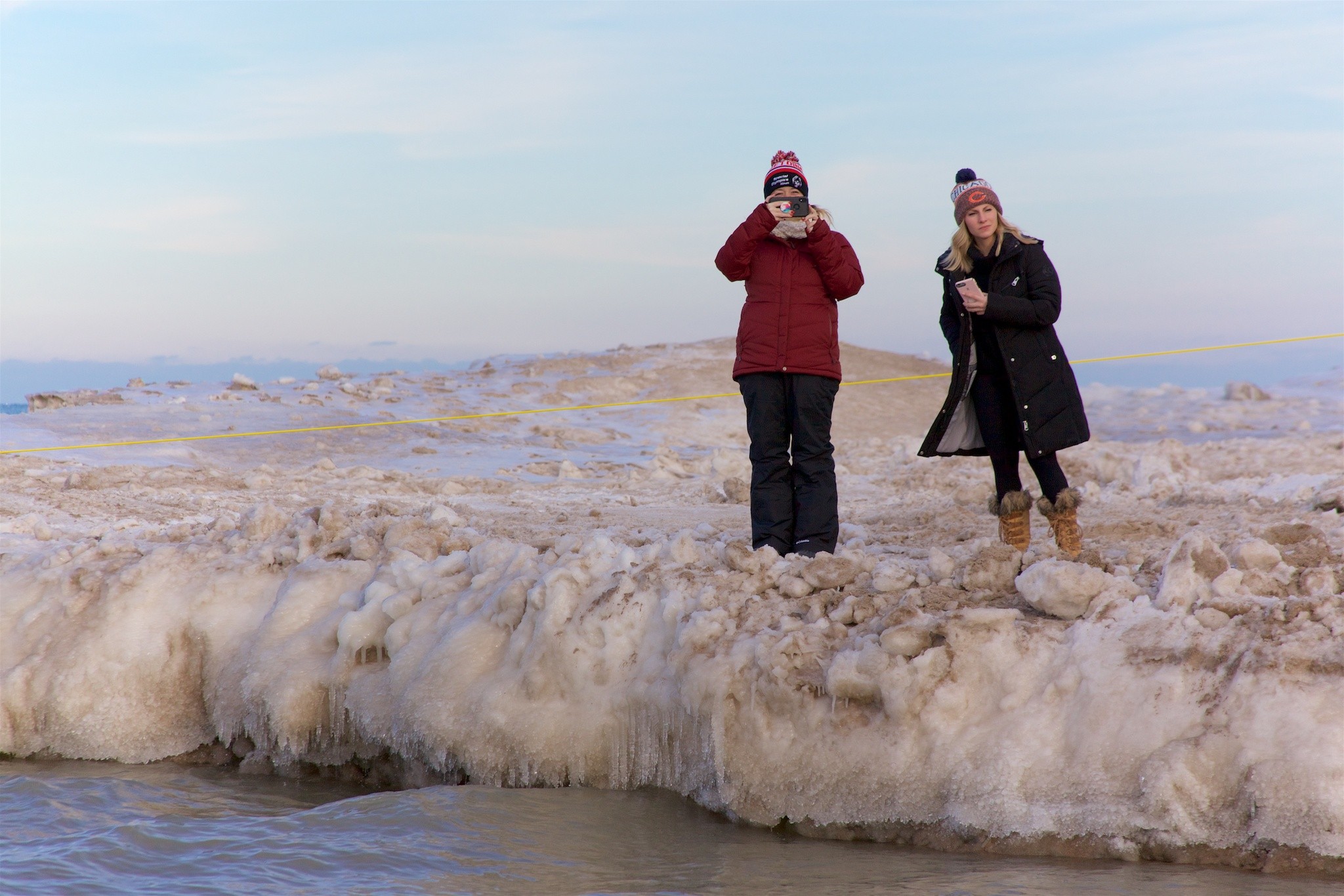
xmin=955 ymin=278 xmax=984 ymax=303
xmin=770 ymin=197 xmax=809 ymax=217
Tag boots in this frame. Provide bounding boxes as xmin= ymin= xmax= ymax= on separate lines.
xmin=986 ymin=488 xmax=1034 ymax=554
xmin=1035 ymin=486 xmax=1083 ymax=560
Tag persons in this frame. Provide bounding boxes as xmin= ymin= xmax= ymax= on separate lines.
xmin=715 ymin=151 xmax=864 ymax=558
xmin=916 ymin=168 xmax=1091 ymax=561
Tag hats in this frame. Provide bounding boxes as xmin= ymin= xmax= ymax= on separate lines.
xmin=765 ymin=150 xmax=808 ymax=201
xmin=951 ymin=168 xmax=1003 ymax=226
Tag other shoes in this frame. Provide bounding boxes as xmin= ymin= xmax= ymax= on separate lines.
xmin=796 ymin=549 xmax=815 ymax=558
